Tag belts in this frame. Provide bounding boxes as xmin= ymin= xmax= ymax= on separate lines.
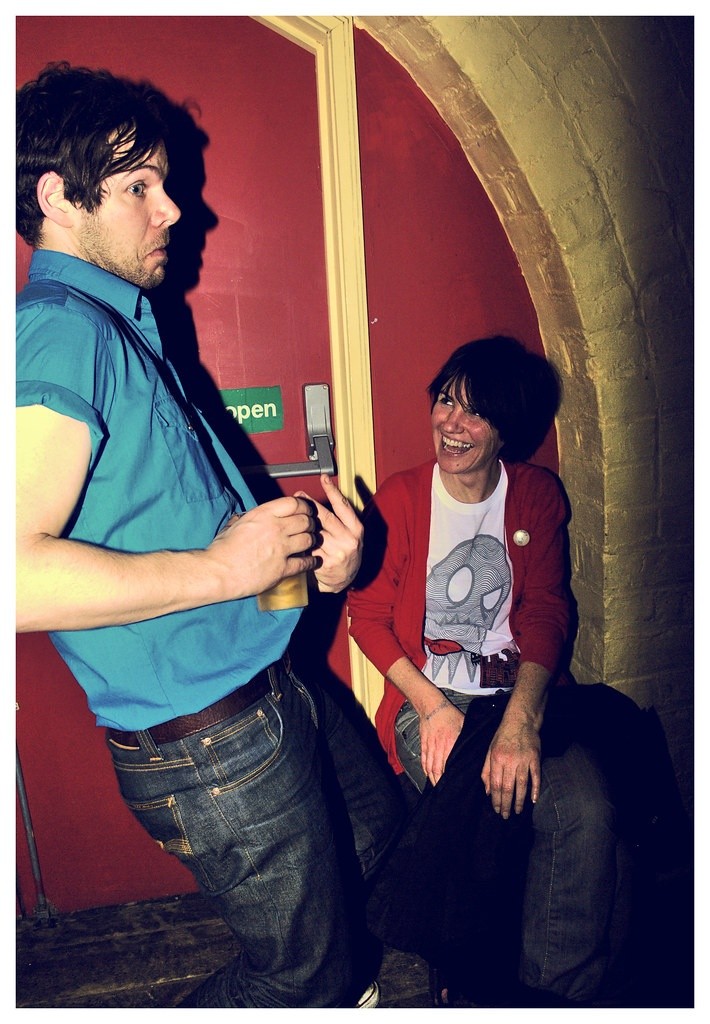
xmin=107 ymin=652 xmax=289 ymax=747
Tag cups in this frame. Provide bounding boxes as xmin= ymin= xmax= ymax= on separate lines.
xmin=258 ymin=550 xmax=308 ymax=612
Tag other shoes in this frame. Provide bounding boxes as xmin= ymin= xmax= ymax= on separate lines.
xmin=349 ymin=980 xmax=380 ymax=1008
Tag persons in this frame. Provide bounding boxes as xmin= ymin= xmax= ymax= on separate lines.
xmin=344 ymin=332 xmax=630 ymax=1008
xmin=16 ymin=62 xmax=397 ymax=1008
